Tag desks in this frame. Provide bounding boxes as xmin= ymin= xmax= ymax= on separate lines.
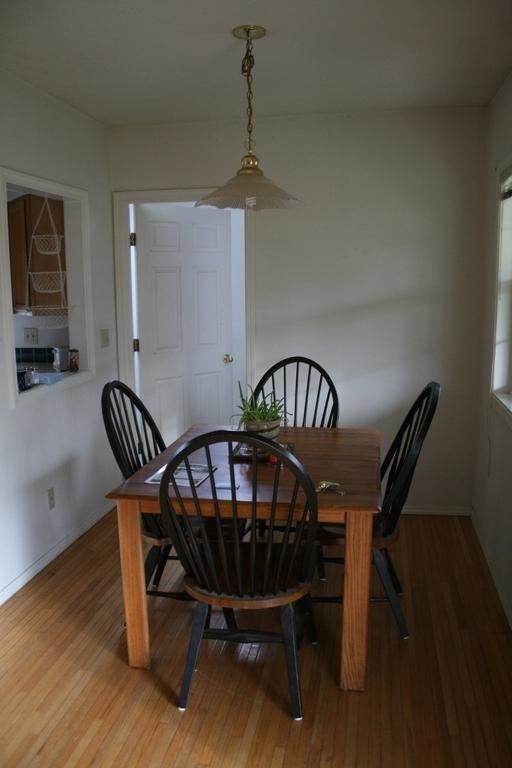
xmin=102 ymin=423 xmax=384 ymax=692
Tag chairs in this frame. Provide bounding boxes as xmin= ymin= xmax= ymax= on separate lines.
xmin=157 ymin=428 xmax=320 ymax=722
xmin=102 ymin=379 xmax=242 ymax=630
xmin=299 ymin=381 xmax=442 ymax=646
xmin=243 ymin=357 xmax=340 ymax=581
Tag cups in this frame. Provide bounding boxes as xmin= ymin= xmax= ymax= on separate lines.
xmin=68 ymin=349 xmax=80 ymax=372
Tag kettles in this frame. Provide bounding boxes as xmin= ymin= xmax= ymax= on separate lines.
xmin=52 ymin=345 xmax=69 ymax=371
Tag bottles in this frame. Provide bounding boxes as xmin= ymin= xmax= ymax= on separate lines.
xmin=24 ymin=366 xmax=40 ymax=387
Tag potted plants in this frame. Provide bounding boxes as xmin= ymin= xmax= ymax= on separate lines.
xmin=227 ymin=379 xmax=291 ymax=452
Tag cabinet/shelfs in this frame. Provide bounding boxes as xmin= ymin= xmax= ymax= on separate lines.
xmin=7 ymin=194 xmax=68 ymax=316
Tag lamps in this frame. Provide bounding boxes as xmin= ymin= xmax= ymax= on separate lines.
xmin=191 ymin=22 xmax=304 ymax=212
